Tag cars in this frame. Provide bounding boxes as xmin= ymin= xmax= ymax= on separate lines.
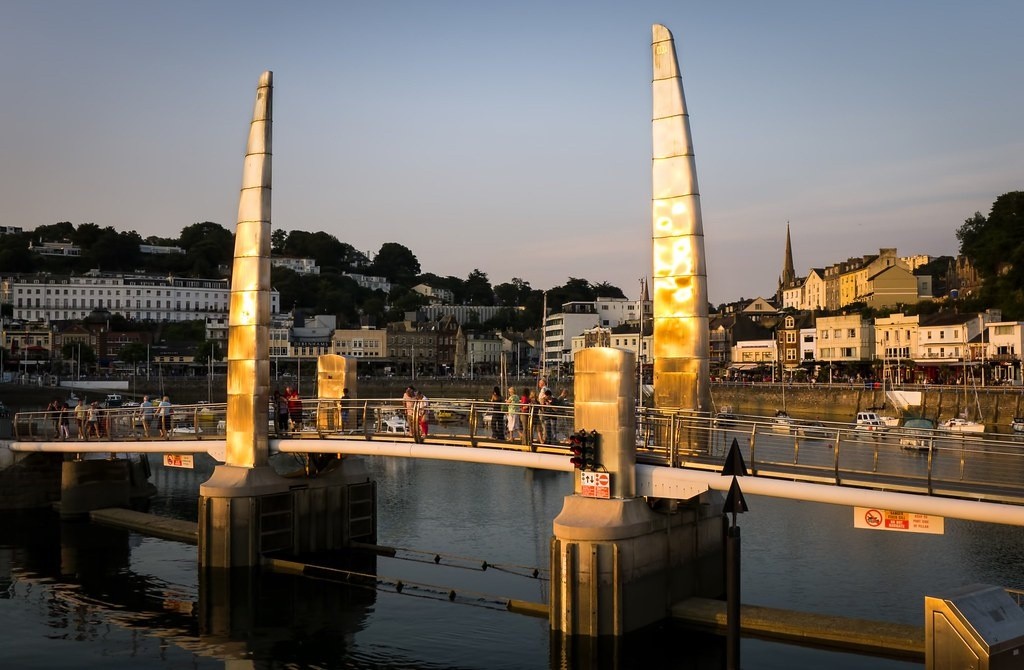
xmin=899 ymin=419 xmax=937 ymax=451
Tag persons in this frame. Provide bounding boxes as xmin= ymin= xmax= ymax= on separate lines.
xmin=276 ymin=384 xmax=302 ymax=436
xmin=47 ymin=400 xmax=70 ymax=438
xmin=138 ymin=395 xmax=154 ymax=437
xmin=833 ymin=370 xmax=880 ymax=383
xmin=74 ymin=399 xmax=104 ymax=439
xmin=154 ymin=395 xmax=172 ymax=438
xmin=734 ymin=371 xmax=738 ymax=381
xmin=403 ymin=386 xmax=429 ymax=437
xmin=340 ymin=388 xmax=354 ymax=435
xmin=491 ymin=378 xmax=569 ymax=443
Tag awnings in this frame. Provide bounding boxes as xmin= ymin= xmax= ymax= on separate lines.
xmin=727 ymin=364 xmax=757 ymax=370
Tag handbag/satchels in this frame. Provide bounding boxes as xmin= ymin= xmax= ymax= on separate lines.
xmin=483 ymin=415 xmax=493 ymax=422
xmin=169 ymin=409 xmax=174 ymax=415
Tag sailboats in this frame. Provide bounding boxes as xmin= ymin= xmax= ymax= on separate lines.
xmin=848 ymin=357 xmax=989 ymax=441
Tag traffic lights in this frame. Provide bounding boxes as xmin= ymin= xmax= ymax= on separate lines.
xmin=568 ymin=430 xmax=599 ymax=471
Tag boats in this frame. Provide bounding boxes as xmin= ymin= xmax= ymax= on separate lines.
xmin=65 ymin=394 xmax=226 ymax=437
xmin=374 ymin=397 xmax=510 ymax=433
xmin=709 ymin=389 xmax=739 ymax=427
xmin=1009 ymin=417 xmax=1024 ymax=432
xmin=770 ymin=361 xmax=835 ymax=439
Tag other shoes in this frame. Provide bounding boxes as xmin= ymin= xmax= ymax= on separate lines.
xmin=160 ymin=434 xmax=164 ymax=437
xmin=421 ymin=433 xmax=426 ymax=438
xmin=506 ymin=438 xmax=514 ymax=443
